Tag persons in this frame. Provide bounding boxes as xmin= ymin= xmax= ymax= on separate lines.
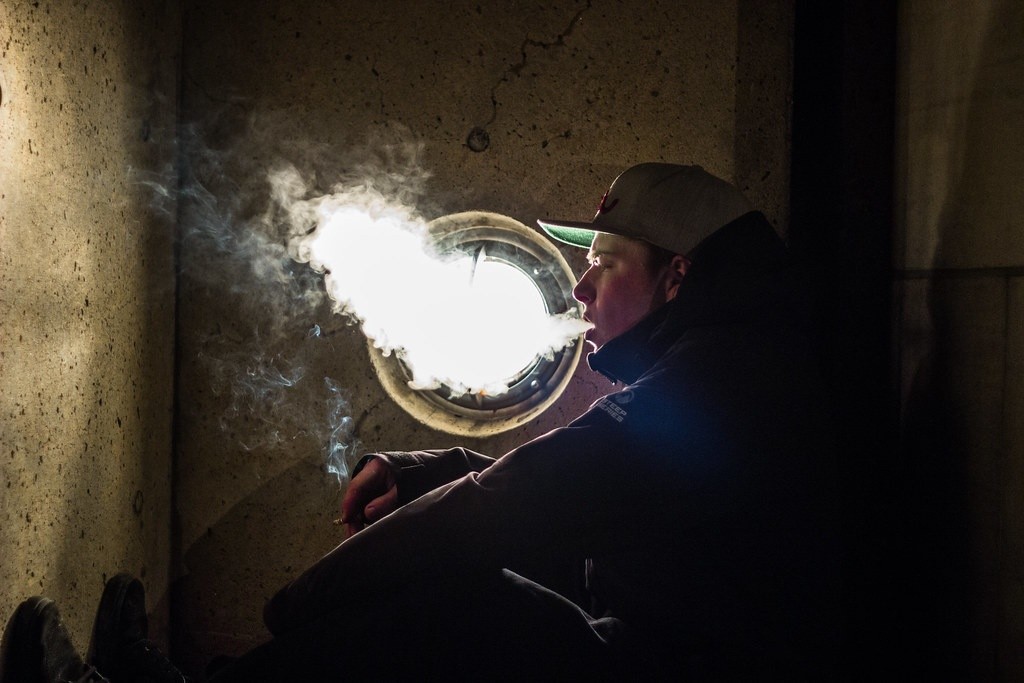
xmin=0 ymin=162 xmax=776 ymax=683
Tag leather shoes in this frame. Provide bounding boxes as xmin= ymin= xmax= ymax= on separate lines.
xmin=4 ymin=573 xmax=198 ymax=683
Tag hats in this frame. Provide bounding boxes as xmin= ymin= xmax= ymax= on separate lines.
xmin=537 ymin=162 xmax=752 ymax=261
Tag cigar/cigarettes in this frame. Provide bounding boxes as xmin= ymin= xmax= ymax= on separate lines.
xmin=332 ymin=517 xmax=345 ymax=527
